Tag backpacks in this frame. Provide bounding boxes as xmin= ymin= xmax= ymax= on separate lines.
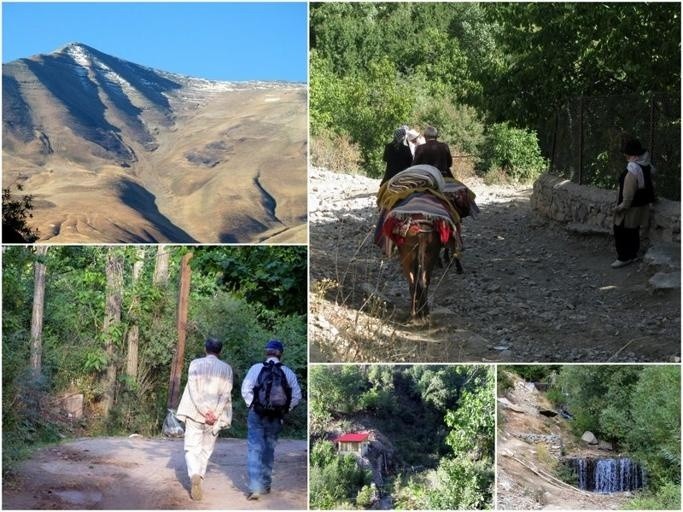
xmin=252 ymin=360 xmax=292 ymax=420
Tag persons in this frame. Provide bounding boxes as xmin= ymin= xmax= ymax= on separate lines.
xmin=379 ymin=129 xmax=413 ymax=188
xmin=175 ymin=336 xmax=235 ymax=501
xmin=414 ymin=126 xmax=454 ymax=177
xmin=241 ymin=340 xmax=303 ymax=500
xmin=611 ymin=138 xmax=656 ymax=269
xmin=400 ymin=124 xmax=426 ymax=160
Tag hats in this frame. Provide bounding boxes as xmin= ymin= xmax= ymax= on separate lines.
xmin=407 ymin=130 xmax=420 ymax=141
xmin=619 ymin=139 xmax=647 ymax=156
xmin=265 ymin=340 xmax=284 ymax=354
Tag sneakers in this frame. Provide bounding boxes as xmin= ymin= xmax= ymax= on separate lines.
xmin=247 ymin=492 xmax=260 ymax=500
xmin=611 ymin=259 xmax=631 ymax=268
xmin=191 ymin=475 xmax=202 ymax=500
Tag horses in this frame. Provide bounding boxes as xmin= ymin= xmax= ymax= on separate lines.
xmin=437 ymin=175 xmax=473 ymax=274
xmin=381 ymin=190 xmax=453 ymax=316
